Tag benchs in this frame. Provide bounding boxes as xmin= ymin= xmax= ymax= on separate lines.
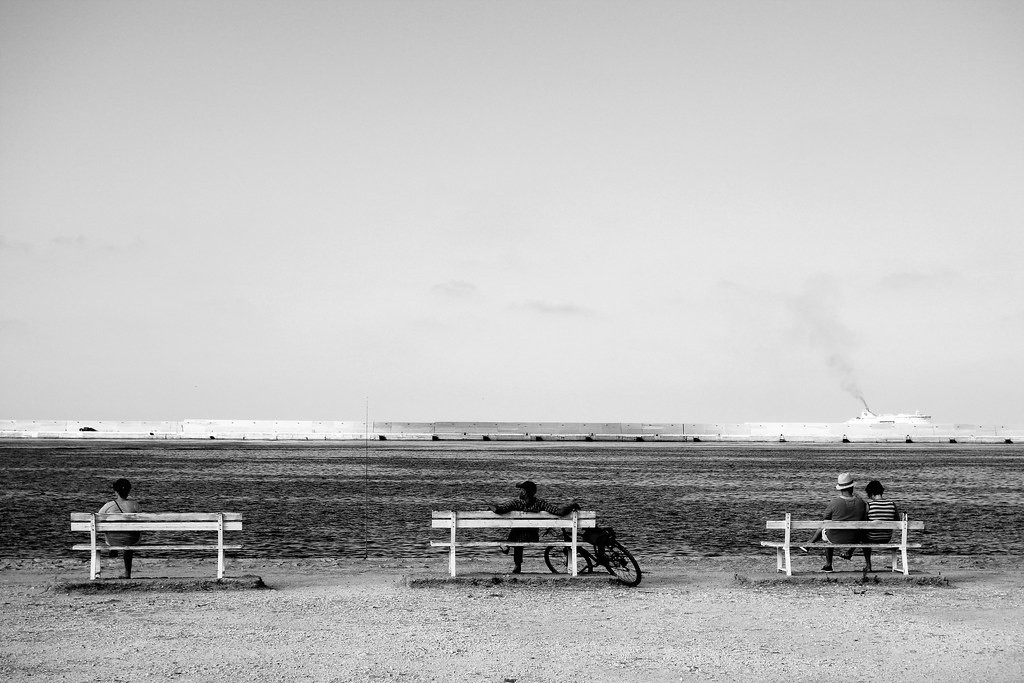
xmin=431 ymin=506 xmax=596 ymax=576
xmin=69 ymin=510 xmax=244 ymax=580
xmin=760 ymin=512 xmax=926 ymax=574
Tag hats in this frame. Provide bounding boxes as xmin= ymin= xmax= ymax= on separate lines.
xmin=516 ymin=481 xmax=537 ymax=494
xmin=836 ymin=472 xmax=857 ymax=490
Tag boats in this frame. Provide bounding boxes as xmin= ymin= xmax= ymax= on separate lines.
xmin=842 ymin=407 xmax=936 ymax=443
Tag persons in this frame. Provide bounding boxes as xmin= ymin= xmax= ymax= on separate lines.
xmin=800 ymin=473 xmax=866 ymax=572
xmin=98 ymin=478 xmax=142 ymax=579
xmin=486 ymin=481 xmax=581 ymax=573
xmin=840 ymin=480 xmax=900 ymax=572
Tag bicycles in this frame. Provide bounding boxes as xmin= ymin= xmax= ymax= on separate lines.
xmin=541 ymin=524 xmax=642 ymax=586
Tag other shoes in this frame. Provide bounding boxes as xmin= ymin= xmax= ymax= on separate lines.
xmin=864 ymin=564 xmax=871 ymax=571
xmin=513 ymin=562 xmax=521 ymax=574
xmin=820 ymin=566 xmax=833 ymax=572
xmin=839 ymin=552 xmax=852 ymax=560
xmin=499 ymin=546 xmax=509 ymax=554
xmin=800 ymin=547 xmax=811 ymax=552
xmin=119 ymin=572 xmax=131 ymax=579
